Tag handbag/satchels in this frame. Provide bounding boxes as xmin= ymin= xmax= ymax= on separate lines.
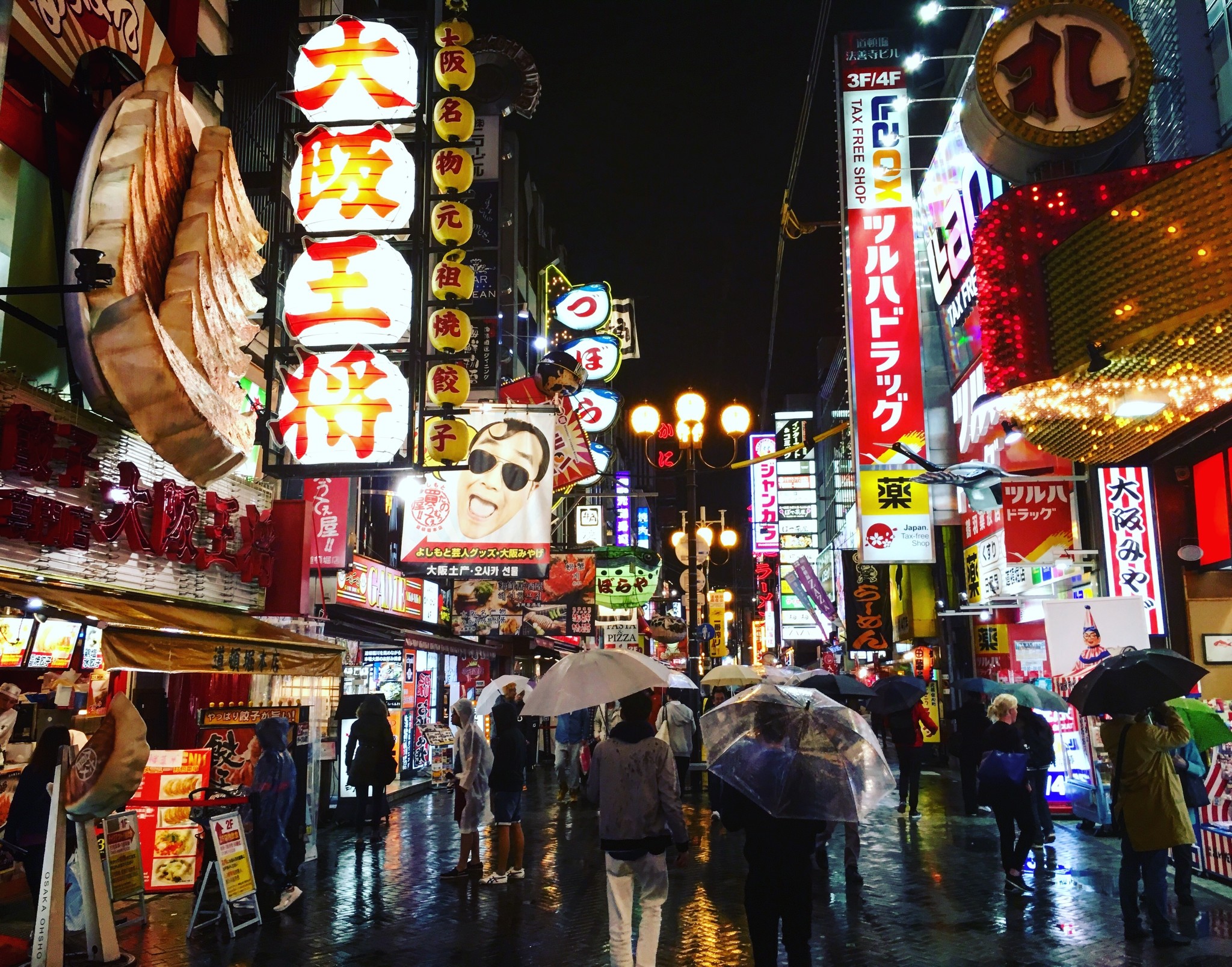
xmin=977 ymin=728 xmax=1027 ymax=786
xmin=654 ymin=705 xmax=670 ymax=746
xmin=64 ymin=849 xmax=85 ymax=930
xmin=579 ymin=744 xmax=592 ymax=776
xmin=1177 ymin=771 xmax=1210 ymax=809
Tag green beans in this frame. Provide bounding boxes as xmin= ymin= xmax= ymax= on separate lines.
xmin=548 ymin=608 xmax=561 ymax=619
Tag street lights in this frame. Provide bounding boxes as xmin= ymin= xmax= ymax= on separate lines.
xmin=672 ymin=526 xmax=739 ymax=698
xmin=630 ymin=389 xmax=754 ymax=798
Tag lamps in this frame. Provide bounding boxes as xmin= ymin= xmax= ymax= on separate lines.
xmin=1054 ymin=557 xmax=1097 ymax=571
xmin=502 ymin=302 xmax=529 ymax=318
xmin=890 ymin=94 xmax=957 ymax=113
xmin=934 ymin=598 xmax=947 ymax=613
xmin=901 ymin=53 xmax=974 ymax=76
xmin=988 ymin=581 xmax=998 ymax=596
xmin=880 ymin=132 xmax=943 ymax=147
xmin=1001 ymin=420 xmax=1021 ymax=444
xmin=872 ymin=165 xmax=928 ymax=179
xmin=503 ymin=334 xmax=548 ymax=350
xmin=1087 ymin=342 xmax=1111 ymax=373
xmin=914 ymin=0 xmax=995 ymax=26
xmin=958 ymin=591 xmax=969 ymax=606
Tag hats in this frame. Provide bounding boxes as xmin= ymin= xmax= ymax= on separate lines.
xmin=0 ymin=683 xmax=21 ymax=700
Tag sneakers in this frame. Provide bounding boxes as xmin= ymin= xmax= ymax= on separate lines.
xmin=479 ymin=871 xmax=508 ymax=883
xmin=233 ymin=897 xmax=254 ymax=909
xmin=467 ymin=861 xmax=483 ymax=871
xmin=506 ymin=867 xmax=525 ymax=879
xmin=439 ymin=867 xmax=469 ymax=878
xmin=274 ymin=885 xmax=302 ymax=912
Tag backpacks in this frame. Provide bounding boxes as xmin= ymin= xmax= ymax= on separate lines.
xmin=1018 ymin=712 xmax=1055 ymax=768
xmin=890 ymin=708 xmax=916 ymax=743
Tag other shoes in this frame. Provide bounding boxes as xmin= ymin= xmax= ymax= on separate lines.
xmin=1005 ymin=872 xmax=1034 ymax=892
xmin=897 ymin=802 xmax=906 ymax=814
xmin=844 ymin=865 xmax=863 ymax=885
xmin=1153 ymin=928 xmax=1180 ymax=940
xmin=1177 ymin=893 xmax=1193 ymax=906
xmin=909 ymin=810 xmax=921 ymax=818
xmin=1044 ymin=833 xmax=1055 ymax=844
xmin=570 ymin=796 xmax=577 ymax=802
xmin=1124 ymin=924 xmax=1144 ymax=938
xmin=556 ymin=787 xmax=568 ymax=801
xmin=711 ymin=811 xmax=721 ymax=823
xmin=1031 ymin=841 xmax=1044 ymax=848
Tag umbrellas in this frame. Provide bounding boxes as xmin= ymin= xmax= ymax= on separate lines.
xmin=476 ymin=675 xmax=529 ymax=715
xmin=524 ymin=648 xmax=669 ymax=715
xmin=951 ymin=649 xmax=1232 ymax=752
xmin=654 ymin=668 xmax=698 ymax=689
xmin=699 ymin=664 xmax=928 ymax=825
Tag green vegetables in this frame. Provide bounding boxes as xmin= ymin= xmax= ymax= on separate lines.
xmin=476 ymin=582 xmax=493 ymax=595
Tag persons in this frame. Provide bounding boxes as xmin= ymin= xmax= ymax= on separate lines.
xmin=248 ymin=719 xmax=310 ymax=911
xmin=556 ymin=682 xmax=733 ymax=967
xmin=0 ymin=682 xmax=24 ymax=765
xmin=1100 ymin=700 xmax=1206 ymax=948
xmin=454 ymin=419 xmax=551 ymax=541
xmin=441 ymin=682 xmax=544 ymax=886
xmin=344 ymin=696 xmax=397 ymax=849
xmin=739 ymin=683 xmax=940 ymax=967
xmin=3 ymin=724 xmax=100 ymax=957
xmin=952 ymin=691 xmax=1057 ymax=894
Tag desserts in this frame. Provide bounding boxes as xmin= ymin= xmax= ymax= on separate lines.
xmin=649 ymin=616 xmax=687 ymax=644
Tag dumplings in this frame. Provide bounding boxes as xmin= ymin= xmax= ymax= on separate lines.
xmin=46 ymin=691 xmax=150 ymax=818
xmin=82 ymin=62 xmax=270 ymax=482
